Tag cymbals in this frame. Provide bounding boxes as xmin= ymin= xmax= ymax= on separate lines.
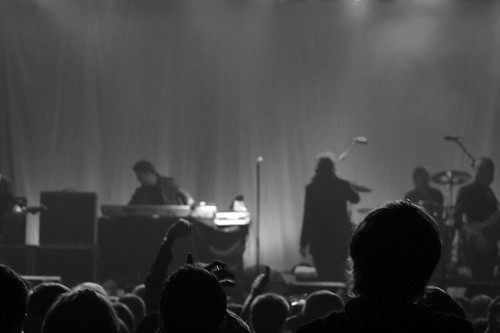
xmin=430 ymin=168 xmax=474 ymax=185
xmin=356 ymin=207 xmax=375 ymax=215
xmin=353 ymin=184 xmax=372 ymax=193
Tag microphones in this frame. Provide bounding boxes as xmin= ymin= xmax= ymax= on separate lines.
xmin=444 ymin=136 xmax=460 ymax=140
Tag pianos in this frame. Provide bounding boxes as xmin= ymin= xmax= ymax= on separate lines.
xmin=100 ymin=203 xmax=217 ymax=220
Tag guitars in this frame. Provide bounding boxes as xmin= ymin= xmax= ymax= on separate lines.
xmin=0 ymin=204 xmax=47 ymax=223
xmin=457 ymin=209 xmax=500 ymax=256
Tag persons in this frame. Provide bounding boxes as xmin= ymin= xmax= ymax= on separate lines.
xmin=128 ymin=160 xmax=184 ymax=205
xmin=0 ymin=198 xmax=500 ymax=333
xmin=452 ymin=157 xmax=499 ymax=281
xmin=404 ymin=165 xmax=445 ymax=215
xmin=299 ymin=156 xmax=359 ymax=280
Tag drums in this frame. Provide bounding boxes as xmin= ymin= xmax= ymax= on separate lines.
xmin=416 ymin=200 xmax=439 ymax=221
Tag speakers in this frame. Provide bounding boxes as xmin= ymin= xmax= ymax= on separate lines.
xmin=32 ymin=190 xmax=99 ymax=289
